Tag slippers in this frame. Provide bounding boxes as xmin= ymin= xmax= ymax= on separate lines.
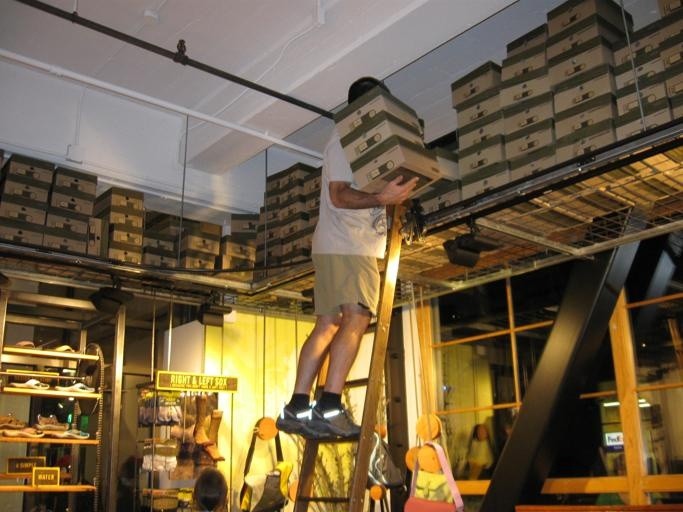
xmin=54 ymin=382 xmax=96 ymax=393
xmin=0 ymin=413 xmax=90 ymax=440
xmin=8 ymin=379 xmax=50 ymax=390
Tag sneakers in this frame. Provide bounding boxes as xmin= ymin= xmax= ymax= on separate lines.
xmin=312 ymin=406 xmax=361 ymax=438
xmin=275 ymin=406 xmax=337 ymax=440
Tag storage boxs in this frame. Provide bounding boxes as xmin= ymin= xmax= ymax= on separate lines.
xmin=0 ymin=148 xmax=257 ymax=283
xmin=334 ymin=85 xmax=461 ymax=215
xmin=257 ymin=162 xmax=324 ymax=281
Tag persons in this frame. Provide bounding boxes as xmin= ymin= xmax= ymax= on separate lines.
xmin=116 ymin=457 xmax=152 ymax=512
xmin=186 ymin=467 xmax=229 ymax=512
xmin=276 ymin=76 xmax=420 ymax=439
xmin=465 ymin=424 xmax=495 ymax=478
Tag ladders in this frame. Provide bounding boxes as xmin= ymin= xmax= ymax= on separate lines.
xmin=293 ymin=203 xmax=409 ymax=512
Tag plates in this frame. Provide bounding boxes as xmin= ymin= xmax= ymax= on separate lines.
xmin=0 ymin=414 xmax=90 ymax=439
xmin=10 ymin=379 xmax=97 ymax=392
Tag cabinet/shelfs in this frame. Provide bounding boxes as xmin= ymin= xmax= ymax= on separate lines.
xmin=0 ymin=269 xmax=109 ymax=512
xmin=135 ymin=382 xmax=220 ymax=512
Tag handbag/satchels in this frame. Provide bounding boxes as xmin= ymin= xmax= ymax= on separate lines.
xmin=465 ymin=424 xmax=495 ymax=468
xmin=366 ymin=431 xmax=404 ymax=490
xmin=239 ymin=461 xmax=293 ymax=512
xmin=404 ymin=496 xmax=457 ymax=512
